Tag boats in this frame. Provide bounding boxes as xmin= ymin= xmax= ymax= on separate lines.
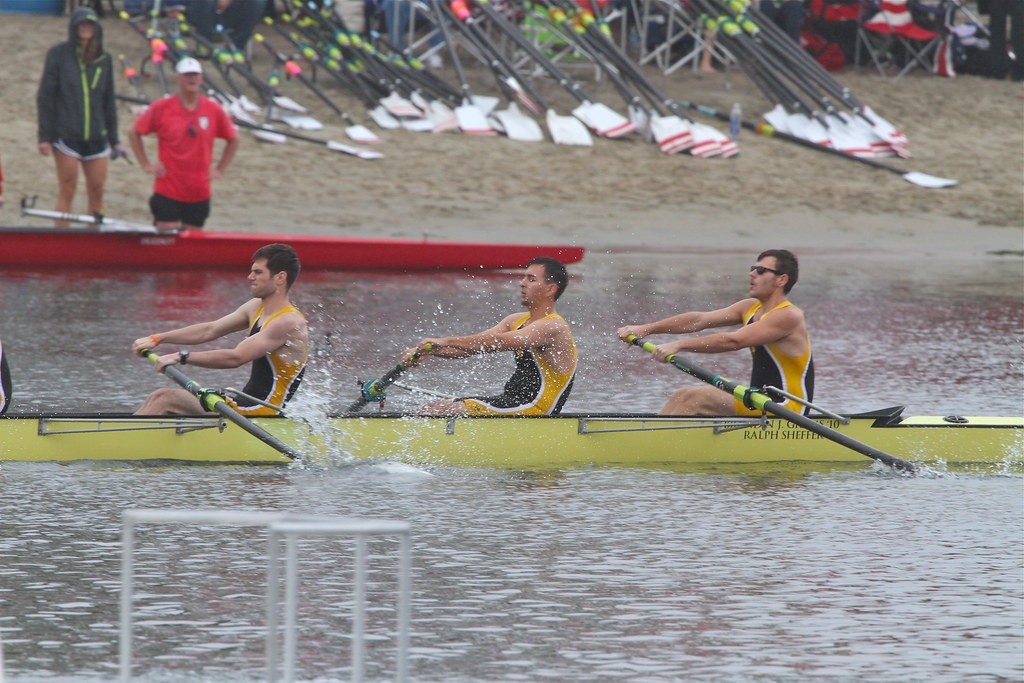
xmin=0 ymin=400 xmax=1024 ymax=477
xmin=0 ymin=220 xmax=587 ymax=277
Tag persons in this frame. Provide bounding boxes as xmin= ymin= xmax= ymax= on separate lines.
xmin=35 ymin=8 xmax=120 ymax=225
xmin=402 ymin=257 xmax=576 ymax=416
xmin=615 ymin=249 xmax=813 ymax=415
xmin=128 ymin=56 xmax=238 ymax=230
xmin=131 ymin=243 xmax=309 ymax=415
xmin=172 ymin=1 xmax=1024 ymax=78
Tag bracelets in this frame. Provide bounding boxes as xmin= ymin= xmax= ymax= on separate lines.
xmin=179 ymin=349 xmax=189 ymax=364
xmin=149 ymin=334 xmax=161 ymax=345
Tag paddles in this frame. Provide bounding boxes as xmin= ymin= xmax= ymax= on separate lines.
xmin=618 ymin=327 xmax=929 ymax=475
xmin=23 ymin=200 xmax=197 ymax=242
xmin=141 ymin=349 xmax=318 ymax=477
xmin=340 ymin=349 xmax=435 ymax=408
xmin=105 ymin=0 xmax=969 ymax=192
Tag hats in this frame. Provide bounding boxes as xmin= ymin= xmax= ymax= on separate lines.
xmin=176 ymin=57 xmax=202 ymax=74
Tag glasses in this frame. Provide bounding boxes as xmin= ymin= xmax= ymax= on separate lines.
xmin=750 ymin=265 xmax=782 ymax=276
